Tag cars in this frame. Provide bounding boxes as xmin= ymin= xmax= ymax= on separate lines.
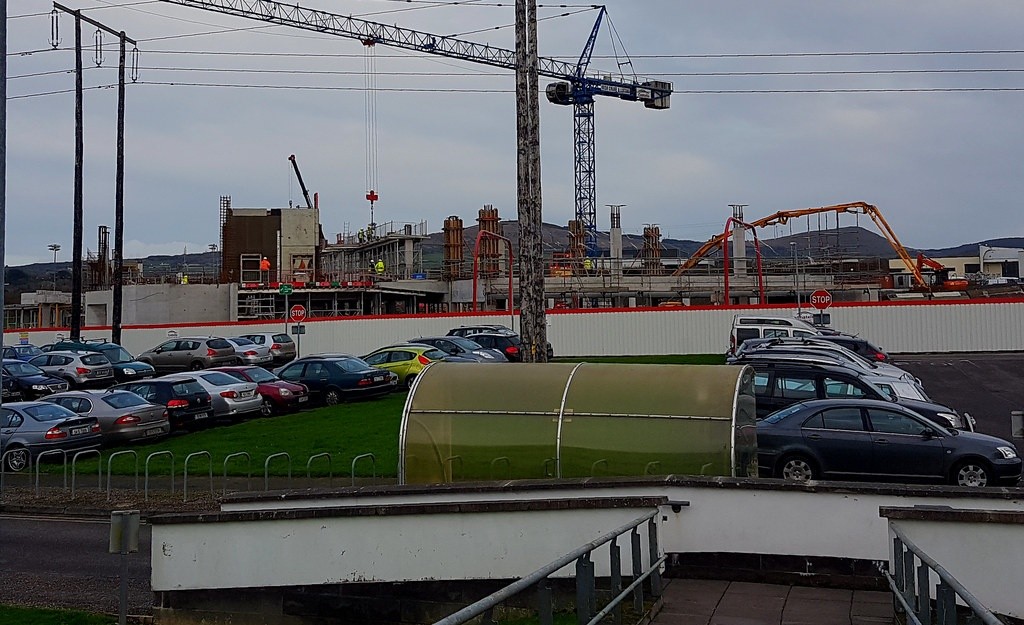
xmin=0 ymin=402 xmax=102 ymax=472
xmin=814 ymin=327 xmax=860 ymax=338
xmin=272 ymin=352 xmax=398 ymax=388
xmin=238 ymin=332 xmax=296 ymax=364
xmin=38 ymin=344 xmax=56 ymax=353
xmin=756 ymin=398 xmax=1023 ymax=486
xmin=275 ymin=358 xmax=391 ymax=405
xmin=33 ymin=389 xmax=170 ymax=450
xmin=356 ymin=342 xmax=490 ymax=364
xmin=199 ymin=367 xmax=310 ymax=416
xmin=3 ymin=344 xmax=45 ymax=362
xmin=222 ymin=337 xmax=273 ymax=366
xmin=2 ymin=358 xmax=69 ymax=400
xmin=362 ymin=346 xmax=447 ymax=389
xmin=156 ymin=371 xmax=263 ymax=420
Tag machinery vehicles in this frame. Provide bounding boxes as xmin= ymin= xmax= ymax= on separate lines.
xmin=658 ymin=199 xmax=937 ymax=307
xmin=912 ymin=252 xmax=942 ymax=291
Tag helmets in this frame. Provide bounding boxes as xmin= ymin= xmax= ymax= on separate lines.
xmin=360 ymin=228 xmax=364 ymax=230
xmin=379 ymin=259 xmax=382 ymax=261
xmin=263 ymin=257 xmax=267 ymax=259
xmin=586 ymin=256 xmax=589 ymax=258
xmin=184 ymin=276 xmax=187 ymax=277
xmin=370 ymin=260 xmax=374 ymax=262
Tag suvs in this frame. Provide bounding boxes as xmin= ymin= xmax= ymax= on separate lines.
xmin=105 ymin=376 xmax=214 ymax=437
xmin=52 ymin=337 xmax=156 ymax=385
xmin=726 ymin=353 xmax=979 ymax=435
xmin=734 ymin=336 xmax=934 ymax=403
xmin=445 ymin=325 xmax=553 ymax=361
xmin=27 ymin=350 xmax=114 ymax=389
xmin=136 ymin=336 xmax=236 ymax=373
xmin=405 ymin=335 xmax=510 ymax=363
xmin=465 ymin=333 xmax=522 ymax=362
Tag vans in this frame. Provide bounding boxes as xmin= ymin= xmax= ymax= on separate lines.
xmin=730 ymin=324 xmax=817 ymax=357
xmin=730 ymin=314 xmax=823 ymax=344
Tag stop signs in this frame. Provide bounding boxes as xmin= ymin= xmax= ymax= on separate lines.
xmin=810 ymin=289 xmax=832 ymax=309
xmin=290 ymin=305 xmax=306 ymax=322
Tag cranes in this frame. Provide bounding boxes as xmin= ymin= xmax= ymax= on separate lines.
xmin=156 ymin=1 xmax=670 ymax=269
xmin=288 ymin=151 xmax=330 ymax=248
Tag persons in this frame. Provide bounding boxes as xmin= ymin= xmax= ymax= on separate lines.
xmin=375 ymin=259 xmax=384 ymax=276
xmin=358 ymin=228 xmax=366 ymax=243
xmin=366 ymin=226 xmax=373 ymax=242
xmin=181 ymin=273 xmax=188 ymax=285
xmin=583 ymin=256 xmax=592 ymax=274
xmin=259 ymin=257 xmax=270 ymax=271
xmin=368 ymin=260 xmax=377 ymax=282
